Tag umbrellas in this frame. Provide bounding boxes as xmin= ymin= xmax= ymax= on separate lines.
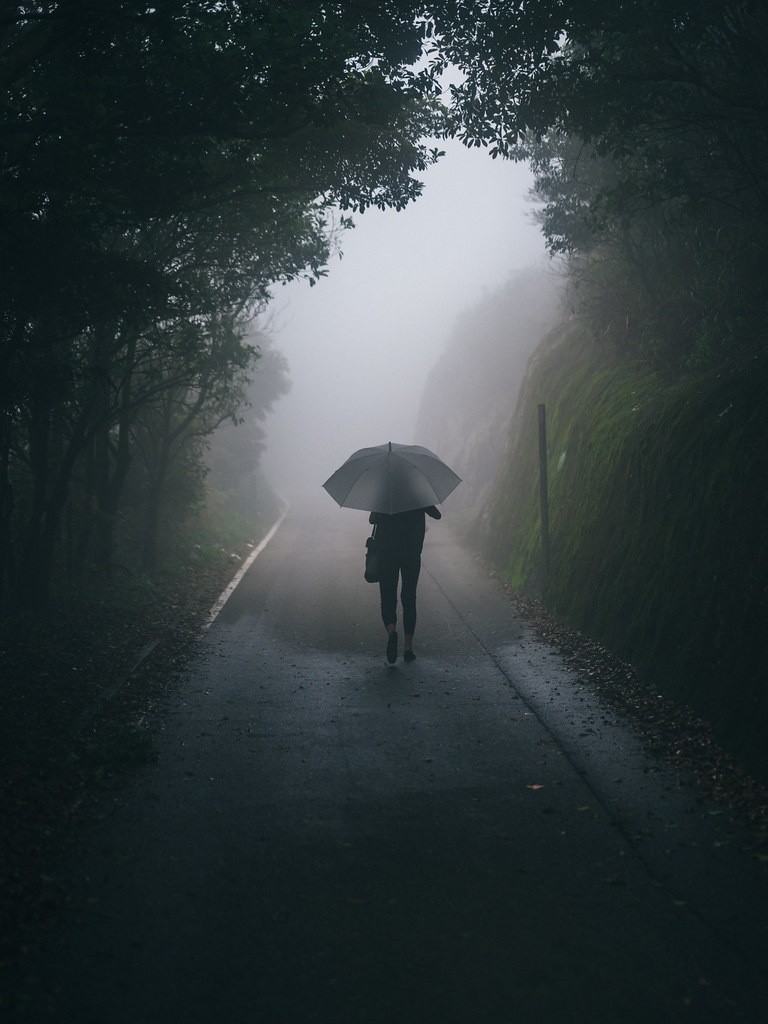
xmin=321 ymin=441 xmax=463 ymax=515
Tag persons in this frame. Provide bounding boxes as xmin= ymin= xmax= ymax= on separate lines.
xmin=369 ymin=473 xmax=442 ymax=664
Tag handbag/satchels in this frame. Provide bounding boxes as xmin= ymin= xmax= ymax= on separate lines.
xmin=363 ymin=538 xmax=381 ymax=584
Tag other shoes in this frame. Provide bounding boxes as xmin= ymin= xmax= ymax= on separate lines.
xmin=386 ymin=632 xmax=398 ymax=664
xmin=404 ymin=650 xmax=416 ymax=662
